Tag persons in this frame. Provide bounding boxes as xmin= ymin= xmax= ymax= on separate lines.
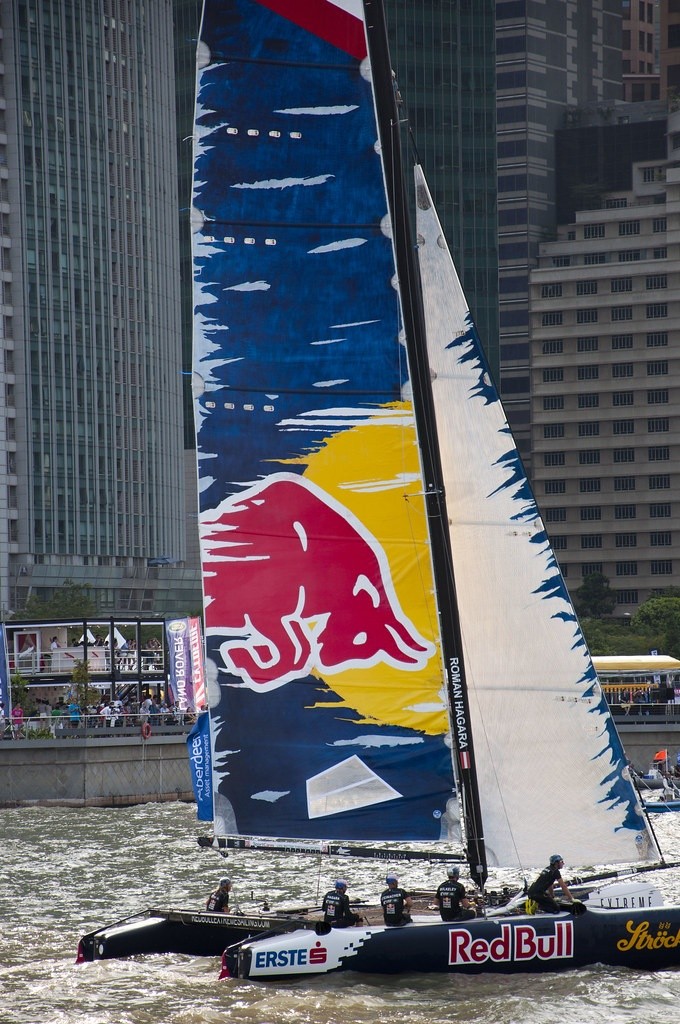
xmin=620 ymin=687 xmax=649 ymax=715
xmin=434 ymin=866 xmax=476 ymax=922
xmin=381 ymin=875 xmax=414 ymax=927
xmin=206 ymin=877 xmax=232 ymax=912
xmin=528 ymin=855 xmax=582 ymax=914
xmin=0 ymin=634 xmax=207 ymax=739
xmin=653 ymin=751 xmax=680 ymax=777
xmin=322 ymin=879 xmax=361 ymax=928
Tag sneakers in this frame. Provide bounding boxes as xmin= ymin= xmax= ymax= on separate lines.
xmin=525 ymin=899 xmax=539 ymax=915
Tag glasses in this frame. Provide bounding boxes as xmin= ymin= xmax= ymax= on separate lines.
xmin=560 ymin=861 xmax=563 ymax=863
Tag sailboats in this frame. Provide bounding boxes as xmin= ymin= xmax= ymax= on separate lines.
xmin=190 ymin=1 xmax=680 ymax=985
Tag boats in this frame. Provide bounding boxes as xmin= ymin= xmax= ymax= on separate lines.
xmin=641 ymin=799 xmax=680 ymax=814
xmin=75 ymin=881 xmax=520 ymax=967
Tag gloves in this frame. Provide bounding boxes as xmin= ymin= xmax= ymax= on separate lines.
xmin=553 ymin=897 xmax=561 ymax=902
xmin=570 ymin=898 xmax=581 ymax=903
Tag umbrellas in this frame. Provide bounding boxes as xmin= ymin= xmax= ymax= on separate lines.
xmin=149 ymin=554 xmax=180 ymax=568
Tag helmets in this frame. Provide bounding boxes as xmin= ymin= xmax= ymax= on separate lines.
xmin=220 ymin=879 xmax=230 ymax=886
xmin=550 ymin=855 xmax=561 ymax=864
xmin=447 ymin=866 xmax=459 ymax=876
xmin=386 ymin=874 xmax=396 ymax=884
xmin=335 ymin=879 xmax=346 ymax=888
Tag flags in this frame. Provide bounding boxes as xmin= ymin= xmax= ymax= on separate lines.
xmin=653 ymin=749 xmax=666 ymax=761
xmin=163 ymin=617 xmax=206 ymax=713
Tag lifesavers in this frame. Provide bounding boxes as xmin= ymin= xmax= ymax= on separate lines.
xmin=141 ymin=722 xmax=152 ymax=739
